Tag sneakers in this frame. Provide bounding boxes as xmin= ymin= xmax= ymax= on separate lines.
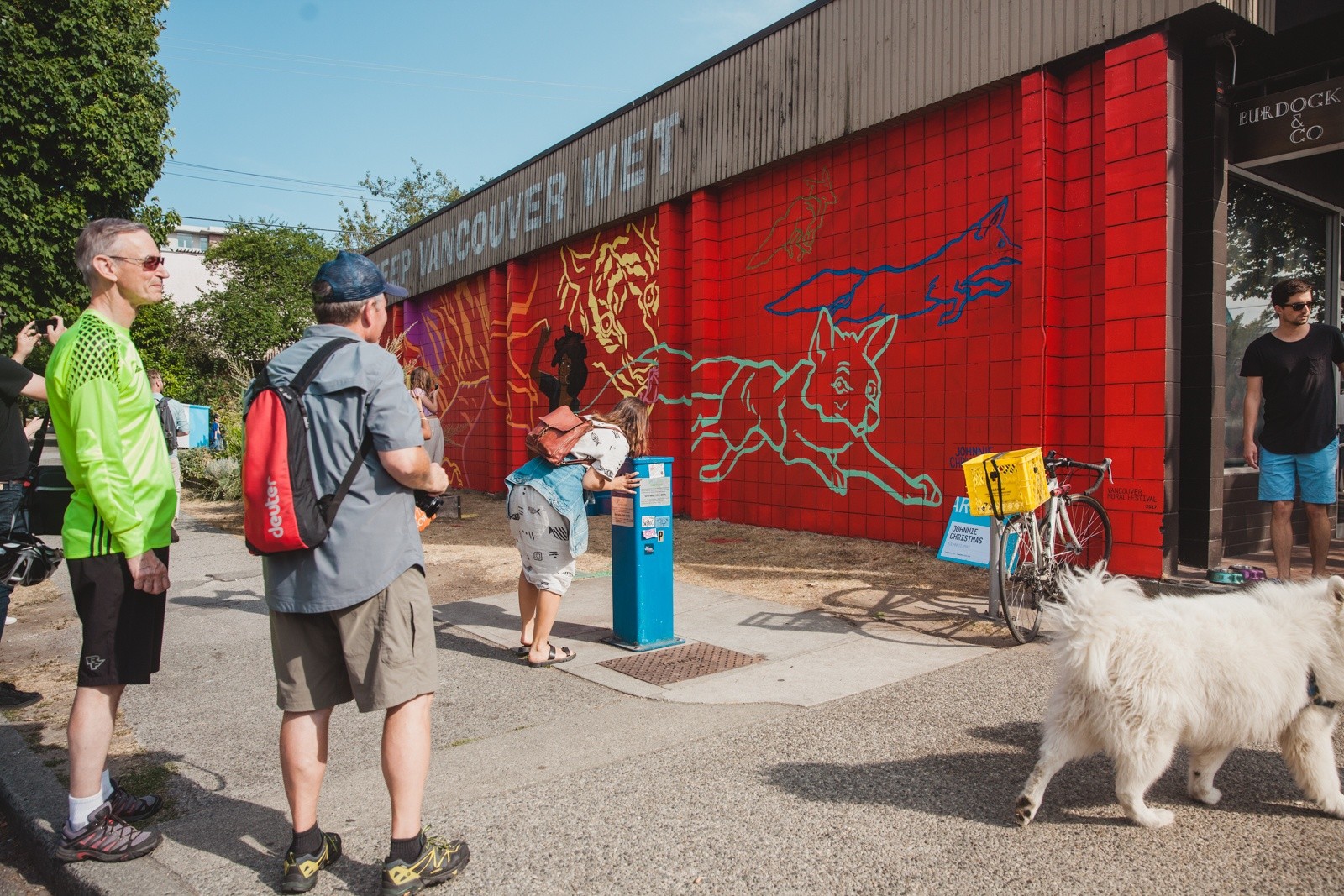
xmin=380 ymin=822 xmax=470 ymax=896
xmin=102 ymin=778 xmax=165 ymax=823
xmin=280 ymin=828 xmax=342 ymax=892
xmin=53 ymin=799 xmax=163 ymax=862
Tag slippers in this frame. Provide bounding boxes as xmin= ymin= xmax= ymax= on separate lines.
xmin=519 ymin=640 xmax=550 ymax=655
xmin=530 ymin=643 xmax=577 ymax=667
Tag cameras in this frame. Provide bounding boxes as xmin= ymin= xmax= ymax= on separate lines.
xmin=413 ymin=488 xmax=443 ymax=519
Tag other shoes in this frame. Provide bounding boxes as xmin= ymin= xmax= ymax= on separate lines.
xmin=0 ymin=681 xmax=43 ymax=710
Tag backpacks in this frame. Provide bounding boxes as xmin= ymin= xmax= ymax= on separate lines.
xmin=524 ymin=404 xmax=630 ymax=467
xmin=154 ymin=397 xmax=178 ymax=450
xmin=240 ymin=336 xmax=376 ymax=559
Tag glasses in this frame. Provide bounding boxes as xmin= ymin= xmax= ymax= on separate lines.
xmin=1283 ymin=300 xmax=1317 ymax=311
xmin=108 ymin=255 xmax=165 ymax=272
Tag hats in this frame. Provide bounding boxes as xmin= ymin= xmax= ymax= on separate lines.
xmin=311 ymin=250 xmax=409 ymax=304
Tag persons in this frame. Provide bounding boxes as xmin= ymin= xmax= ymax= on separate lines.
xmin=241 ymin=250 xmax=471 ymax=896
xmin=0 ymin=315 xmax=68 ymax=709
xmin=504 ymin=397 xmax=649 ymax=667
xmin=210 ymin=413 xmax=221 ymax=450
xmin=145 ymin=370 xmax=189 ymax=543
xmin=1240 ymin=280 xmax=1344 ymax=582
xmin=44 ymin=220 xmax=177 ymax=862
xmin=408 ymin=367 xmax=444 ymax=466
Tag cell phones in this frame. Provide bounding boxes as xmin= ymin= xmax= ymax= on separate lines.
xmin=435 ymin=383 xmax=438 ymax=390
xmin=34 ymin=319 xmax=57 ymax=335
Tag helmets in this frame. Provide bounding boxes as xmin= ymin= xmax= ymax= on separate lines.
xmin=0 ymin=529 xmax=65 ymax=587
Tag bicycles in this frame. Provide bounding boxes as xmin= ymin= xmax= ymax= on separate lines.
xmin=992 ymin=447 xmax=1117 ymax=644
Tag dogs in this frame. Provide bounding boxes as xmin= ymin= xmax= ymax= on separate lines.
xmin=1009 ymin=557 xmax=1344 ymax=830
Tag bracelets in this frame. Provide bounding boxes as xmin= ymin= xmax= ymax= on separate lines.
xmin=420 ymin=418 xmax=427 ymax=419
xmin=419 ymin=410 xmax=424 ymax=413
